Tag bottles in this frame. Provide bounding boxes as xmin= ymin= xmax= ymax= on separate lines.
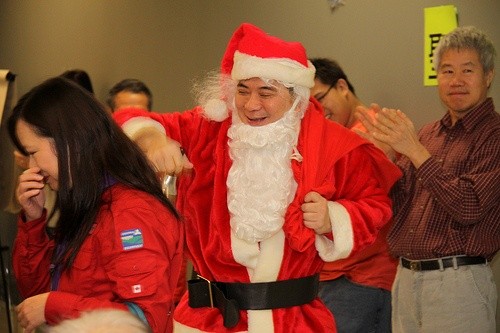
xmin=159 ymin=147 xmax=186 ymax=205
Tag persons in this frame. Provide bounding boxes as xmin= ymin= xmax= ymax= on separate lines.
xmin=111 ymin=22 xmax=400 ymax=333
xmin=351 ymin=25 xmax=500 ymax=333
xmin=8 ymin=69 xmax=187 ymax=333
xmin=309 ymin=57 xmax=404 ymax=333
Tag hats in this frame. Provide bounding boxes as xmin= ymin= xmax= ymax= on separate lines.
xmin=205 ymin=22 xmax=316 ymax=122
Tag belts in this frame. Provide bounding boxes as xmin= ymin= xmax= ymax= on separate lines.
xmin=400 ymin=256 xmax=489 ymax=272
xmin=188 ymin=268 xmax=319 ymax=328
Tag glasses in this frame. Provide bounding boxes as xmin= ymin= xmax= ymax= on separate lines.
xmin=315 ymin=84 xmax=335 ymax=104
